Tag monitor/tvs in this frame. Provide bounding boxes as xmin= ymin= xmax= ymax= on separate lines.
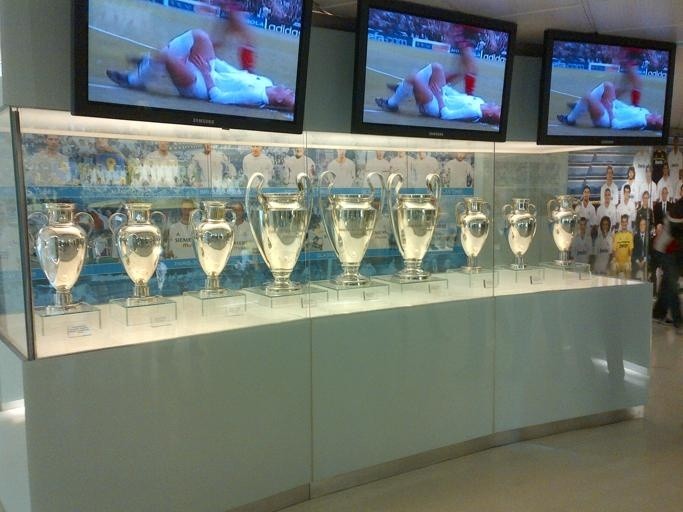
xmin=69 ymin=0 xmax=313 ymax=134
xmin=536 ymin=29 xmax=678 ymax=149
xmin=350 ymin=1 xmax=518 ymax=143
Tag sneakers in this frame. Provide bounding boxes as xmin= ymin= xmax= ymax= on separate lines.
xmin=373 ymin=95 xmax=401 ymax=113
xmin=105 ymin=67 xmax=148 ymax=88
xmin=556 ymin=111 xmax=579 ymax=128
xmin=386 ymin=82 xmax=399 ymax=94
xmin=651 ymin=313 xmax=683 ymax=334
xmin=567 ymin=102 xmax=578 ymax=109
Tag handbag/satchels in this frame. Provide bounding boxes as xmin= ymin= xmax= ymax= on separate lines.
xmin=652 ymin=228 xmax=677 ymax=254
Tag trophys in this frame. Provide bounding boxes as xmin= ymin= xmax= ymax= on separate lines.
xmin=318 ymin=169 xmax=386 ymax=287
xmin=26 ymin=200 xmax=96 ymax=311
xmin=107 ymin=202 xmax=166 ymax=303
xmin=243 ymin=170 xmax=315 ymax=292
xmin=386 ymin=173 xmax=442 ymax=281
xmin=546 ymin=194 xmax=581 ymax=266
xmin=188 ymin=199 xmax=239 ymax=296
xmin=454 ymin=195 xmax=490 ymax=270
xmin=499 ymin=195 xmax=540 ymax=269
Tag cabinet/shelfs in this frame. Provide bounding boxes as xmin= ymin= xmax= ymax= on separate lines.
xmin=0 ymin=105 xmax=653 ymax=512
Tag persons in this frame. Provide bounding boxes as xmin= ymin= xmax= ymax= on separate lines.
xmin=445 ymin=151 xmax=471 ymax=187
xmin=282 ymin=147 xmax=315 ymax=187
xmin=570 ymin=135 xmax=683 ymax=332
xmin=187 ymin=141 xmax=237 ymax=187
xmin=141 ymin=141 xmax=178 ymax=187
xmin=194 ymin=1 xmax=283 ymax=73
xmin=26 ymin=135 xmax=71 ymax=185
xmin=328 ymin=149 xmax=356 ymax=187
xmin=105 ymin=27 xmax=295 ymax=110
xmin=366 ymin=150 xmax=439 ymax=187
xmin=555 ymin=81 xmax=664 ymax=130
xmin=601 ymin=45 xmax=660 ymax=109
xmin=431 ymin=21 xmax=496 ymax=96
xmin=243 ymin=145 xmax=274 ymax=187
xmin=373 ymin=62 xmax=501 ymax=123
